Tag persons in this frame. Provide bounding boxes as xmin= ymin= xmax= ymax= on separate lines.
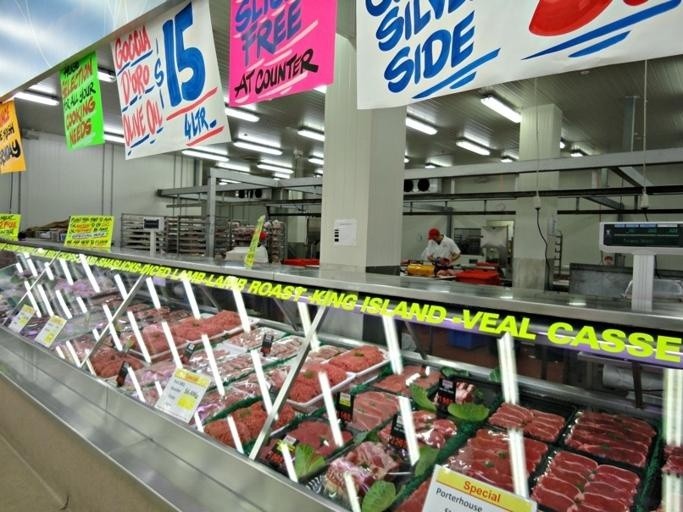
xmin=427 ymin=228 xmax=461 ymax=276
xmin=604 ymin=256 xmax=613 ymax=265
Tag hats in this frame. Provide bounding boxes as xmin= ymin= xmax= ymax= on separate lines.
xmin=428 ymin=228 xmax=439 ymax=239
xmin=260 ymin=231 xmax=265 ymax=240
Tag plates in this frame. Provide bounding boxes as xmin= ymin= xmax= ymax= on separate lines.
xmin=220 ymin=326 xmax=286 ymax=350
xmin=120 ymin=326 xmax=190 ymax=358
xmin=369 ymin=358 xmax=442 ymax=404
xmin=248 ymin=416 xmax=362 ymax=483
xmin=50 ymin=332 xmax=144 ymax=378
xmin=184 ymin=345 xmax=251 ymax=367
xmin=307 ymin=344 xmax=347 ymax=363
xmin=183 ymin=385 xmax=254 ymax=424
xmin=427 ymin=376 xmax=498 ymax=416
xmin=267 ymin=366 xmax=355 ymax=414
xmin=300 ymin=442 xmax=425 ymax=510
xmin=257 ymin=335 xmax=305 ymax=360
xmin=530 ymin=448 xmax=647 ymax=511
xmin=111 ymin=359 xmax=191 ymax=392
xmin=322 ymin=345 xmax=391 ymax=376
xmin=132 ymin=385 xmax=167 ymax=405
xmin=118 ymin=306 xmax=169 ymax=323
xmin=284 ymin=355 xmax=325 ymax=372
xmin=169 ymin=311 xmax=257 ymax=343
xmin=206 ymin=395 xmax=297 ymax=453
xmin=484 ymin=388 xmax=575 ymax=444
xmin=323 ymin=386 xmax=414 ymax=430
xmin=443 ymin=426 xmax=549 ymax=490
xmin=557 ymin=406 xmax=660 ymax=472
xmin=231 ymin=376 xmax=272 ymax=395
xmin=196 ymin=353 xmax=276 ymax=384
xmin=370 ymin=406 xmax=461 ymax=447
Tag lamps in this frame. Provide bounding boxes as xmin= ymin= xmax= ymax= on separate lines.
xmin=104 ymin=131 xmax=125 ymax=143
xmin=98 ymin=67 xmax=117 ymax=83
xmin=297 ymin=126 xmax=325 ymax=142
xmin=481 ymin=97 xmax=522 ymax=123
xmin=559 ymin=137 xmax=566 ymax=148
xmin=425 ymin=162 xmax=442 ymax=169
xmin=215 ymin=162 xmax=249 ymax=173
xmin=272 ymin=172 xmax=290 ymax=179
xmin=308 ymin=155 xmax=324 ymax=165
xmin=233 ymin=139 xmax=283 ymax=156
xmin=256 ymin=162 xmax=293 ymax=175
xmin=534 ymin=188 xmax=541 ymax=210
xmin=225 ymin=103 xmax=260 ymax=123
xmin=640 ymin=192 xmax=649 ymax=208
xmin=570 ymin=149 xmax=588 ymax=157
xmin=220 ymin=179 xmax=241 ymax=184
xmin=456 ymin=139 xmax=491 ymax=156
xmin=406 ymin=114 xmax=438 ymax=135
xmin=181 ymin=150 xmax=229 ymax=162
xmin=314 ymin=169 xmax=324 ymax=175
xmin=404 ymin=155 xmax=409 ymax=164
xmin=501 ymin=156 xmax=516 ymax=162
xmin=15 ymin=92 xmax=60 ymax=106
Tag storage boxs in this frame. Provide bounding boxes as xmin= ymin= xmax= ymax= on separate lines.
xmin=456 ymin=269 xmax=499 ymax=285
xmin=448 ymin=329 xmax=494 ymax=351
xmin=283 ymin=259 xmax=319 ymax=266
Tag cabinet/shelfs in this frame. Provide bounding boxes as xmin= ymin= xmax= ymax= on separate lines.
xmin=0 ymin=237 xmax=683 ymax=512
